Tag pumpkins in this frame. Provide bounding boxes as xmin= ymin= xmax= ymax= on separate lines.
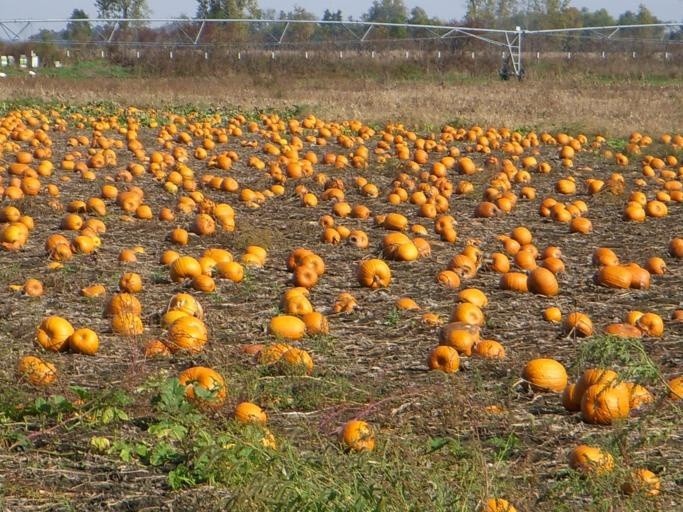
xmin=0 ymin=106 xmax=683 ymax=512
